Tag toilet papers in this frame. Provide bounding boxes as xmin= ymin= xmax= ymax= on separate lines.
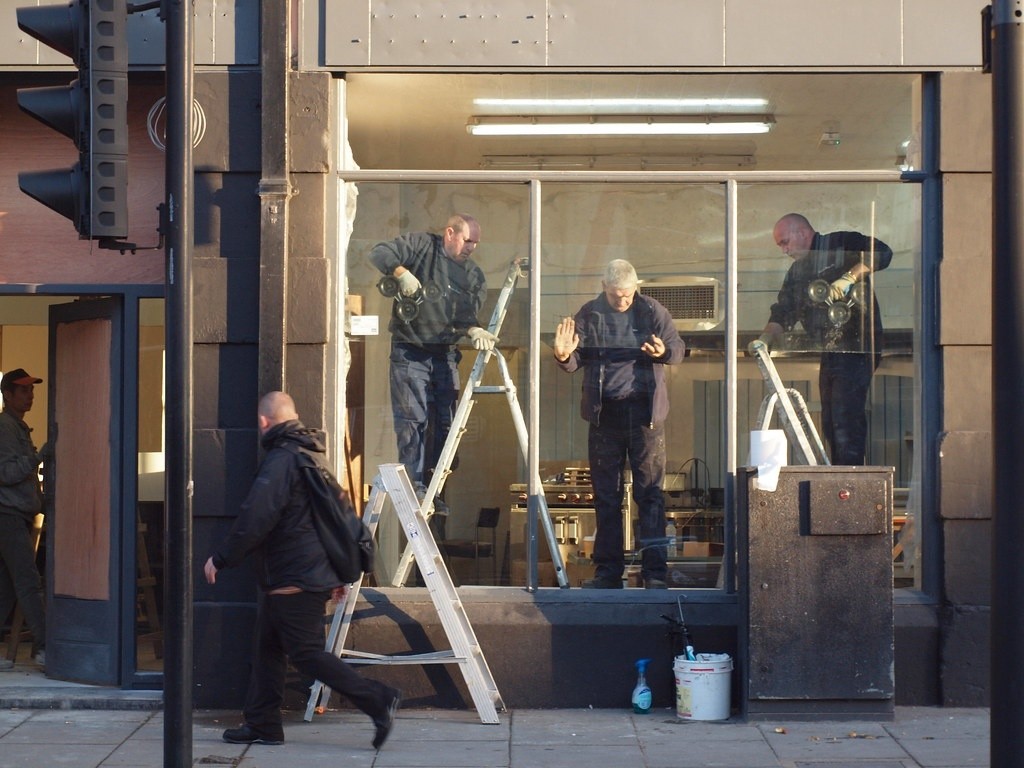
xmin=750 ymin=429 xmax=788 ymax=492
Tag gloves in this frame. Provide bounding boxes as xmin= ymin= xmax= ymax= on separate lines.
xmin=747 ymin=339 xmax=768 ymax=356
xmin=829 ymin=275 xmax=853 ymax=301
xmin=397 ymin=270 xmax=422 ymax=297
xmin=470 ymin=329 xmax=501 ymax=350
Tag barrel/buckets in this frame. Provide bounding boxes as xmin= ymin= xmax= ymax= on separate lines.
xmin=673 ymin=653 xmax=732 ymax=720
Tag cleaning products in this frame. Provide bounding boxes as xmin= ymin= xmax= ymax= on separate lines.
xmin=631 ymin=658 xmax=654 ymax=716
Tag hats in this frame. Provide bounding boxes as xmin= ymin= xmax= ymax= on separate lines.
xmin=0 ymin=369 xmax=43 ymax=392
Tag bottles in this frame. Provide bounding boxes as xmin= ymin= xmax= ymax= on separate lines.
xmin=682 ymin=527 xmax=690 ymax=541
xmin=665 ymin=521 xmax=677 ymax=557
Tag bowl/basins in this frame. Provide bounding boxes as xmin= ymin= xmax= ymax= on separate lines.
xmin=708 ymin=488 xmax=724 ymax=505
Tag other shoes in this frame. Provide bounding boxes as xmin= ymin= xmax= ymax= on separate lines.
xmin=582 ymin=577 xmax=624 ymax=590
xmin=32 ymin=650 xmax=45 ymax=667
xmin=645 ymin=578 xmax=668 ymax=589
xmin=0 ymin=660 xmax=13 ymax=669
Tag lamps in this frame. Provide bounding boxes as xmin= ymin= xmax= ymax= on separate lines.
xmin=467 ymin=117 xmax=775 ymax=134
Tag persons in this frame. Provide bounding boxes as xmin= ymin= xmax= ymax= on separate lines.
xmin=370 ymin=214 xmax=500 ymax=517
xmin=0 ymin=368 xmax=50 ymax=668
xmin=204 ymin=390 xmax=402 ymax=750
xmin=551 ymin=259 xmax=686 ymax=589
xmin=746 ymin=212 xmax=894 ymax=468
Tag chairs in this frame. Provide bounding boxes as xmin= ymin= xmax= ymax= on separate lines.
xmin=445 ymin=507 xmax=500 ymax=584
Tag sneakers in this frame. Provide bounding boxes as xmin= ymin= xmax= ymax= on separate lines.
xmin=413 ymin=485 xmax=435 ymax=516
xmin=434 ymin=497 xmax=449 ymax=516
xmin=373 ymin=686 xmax=402 ymax=748
xmin=223 ymin=726 xmax=284 ymax=745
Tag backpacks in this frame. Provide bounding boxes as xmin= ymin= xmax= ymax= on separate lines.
xmin=278 ymin=442 xmax=379 ymax=583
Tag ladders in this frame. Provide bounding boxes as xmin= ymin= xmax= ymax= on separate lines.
xmin=390 ymin=252 xmax=571 ymax=589
xmin=304 ymin=466 xmax=508 ymax=725
xmin=750 ymin=341 xmax=833 ymax=465
